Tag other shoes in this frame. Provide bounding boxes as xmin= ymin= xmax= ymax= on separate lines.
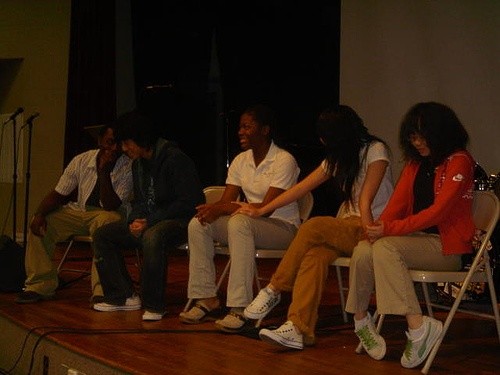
xmin=16 ymin=291 xmax=56 ymax=304
xmin=93 ymin=295 xmax=105 ymax=303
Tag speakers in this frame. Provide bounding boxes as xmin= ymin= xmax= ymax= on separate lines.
xmin=0 ymin=234 xmax=27 ymax=294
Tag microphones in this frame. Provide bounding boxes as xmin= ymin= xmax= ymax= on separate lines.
xmin=20 ymin=112 xmax=39 ymax=128
xmin=4 ymin=107 xmax=24 ymax=124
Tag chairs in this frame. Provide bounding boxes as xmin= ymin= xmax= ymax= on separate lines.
xmin=57 ymin=186 xmax=500 ymax=375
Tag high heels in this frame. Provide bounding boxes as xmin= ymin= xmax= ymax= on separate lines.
xmin=179 ymin=304 xmax=222 ymax=324
xmin=216 ymin=312 xmax=258 ymax=334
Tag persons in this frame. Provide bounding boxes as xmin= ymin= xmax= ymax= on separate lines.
xmin=179 ymin=107 xmax=301 ymax=334
xmin=18 ymin=120 xmax=133 ymax=306
xmin=91 ymin=115 xmax=205 ymax=323
xmin=345 ymin=101 xmax=476 ymax=368
xmin=229 ymin=106 xmax=395 ymax=350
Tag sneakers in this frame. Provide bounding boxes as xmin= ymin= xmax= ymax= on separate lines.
xmin=243 ymin=285 xmax=282 ymax=320
xmin=142 ymin=310 xmax=163 ymax=321
xmin=353 ymin=312 xmax=387 ymax=360
xmin=93 ymin=295 xmax=141 ymax=312
xmin=259 ymin=320 xmax=303 ymax=350
xmin=401 ymin=315 xmax=443 ymax=369
